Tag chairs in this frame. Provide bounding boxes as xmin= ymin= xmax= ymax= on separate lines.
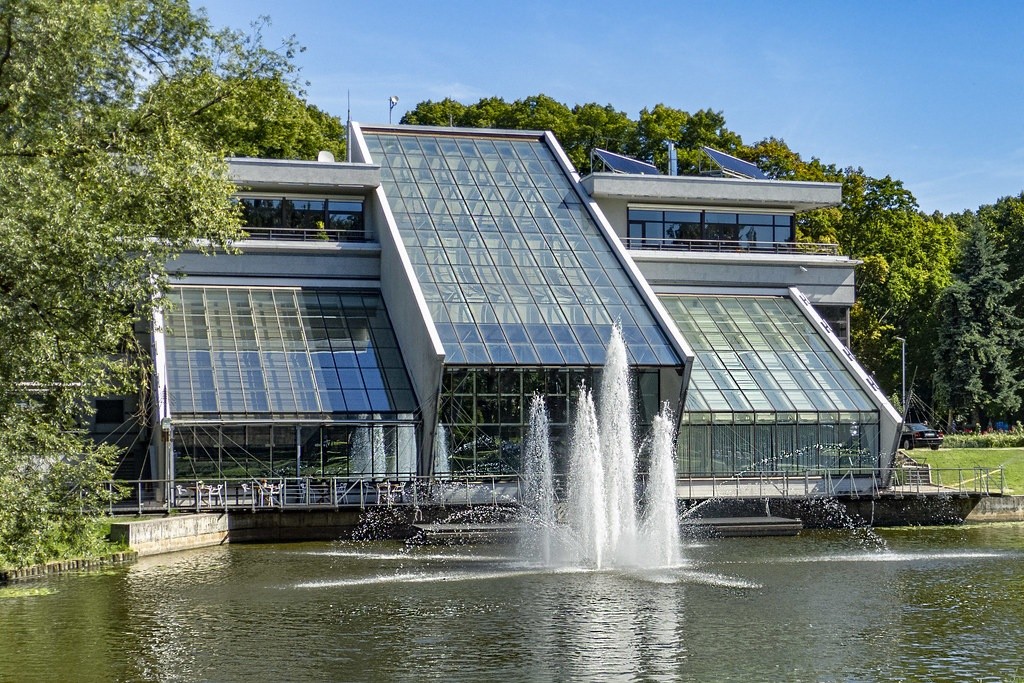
xmin=299 ymin=483 xmax=348 ymax=505
xmin=175 ymin=484 xmax=224 ymax=508
xmin=241 ymin=483 xmax=284 ymax=506
xmin=363 ymin=482 xmax=408 ymax=504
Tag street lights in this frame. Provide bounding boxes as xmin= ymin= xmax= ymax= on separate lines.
xmin=897 ymin=337 xmax=905 ymax=423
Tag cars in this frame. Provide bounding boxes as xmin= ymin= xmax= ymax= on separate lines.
xmin=900 ymin=424 xmax=943 ymax=450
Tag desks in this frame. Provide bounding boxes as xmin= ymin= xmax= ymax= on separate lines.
xmin=249 ymin=486 xmax=271 ymax=507
xmin=310 ymin=485 xmax=330 ymax=503
xmin=185 ymin=486 xmax=209 ymax=507
xmin=380 ymin=484 xmax=399 ymax=504
